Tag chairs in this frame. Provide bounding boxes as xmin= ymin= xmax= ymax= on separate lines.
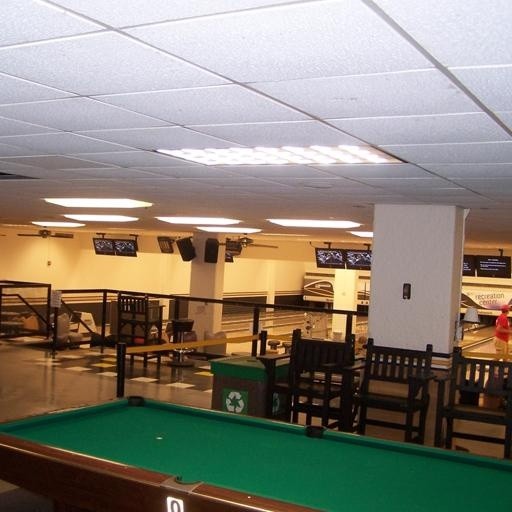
xmin=117 ymin=293 xmax=166 ymax=368
xmin=433 ymin=347 xmax=512 ymax=459
xmin=344 ymin=338 xmax=437 ymax=444
xmin=256 ymin=330 xmax=352 ymax=432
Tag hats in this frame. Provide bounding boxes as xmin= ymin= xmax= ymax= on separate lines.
xmin=501 ymin=305 xmax=510 ymax=311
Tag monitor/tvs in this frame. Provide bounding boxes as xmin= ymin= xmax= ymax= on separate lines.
xmin=346 ymin=250 xmax=372 ymax=269
xmin=114 ymin=239 xmax=136 ymax=256
xmin=226 ymin=241 xmax=241 ymax=255
xmin=316 ymin=248 xmax=345 ymax=268
xmin=93 ymin=238 xmax=115 ymax=255
xmin=477 ymin=256 xmax=511 ymax=278
xmin=158 ymin=240 xmax=173 ymax=253
xmin=462 ymin=255 xmax=475 ymax=276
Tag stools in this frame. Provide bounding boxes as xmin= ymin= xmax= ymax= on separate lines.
xmin=166 ymin=319 xmax=195 ymax=367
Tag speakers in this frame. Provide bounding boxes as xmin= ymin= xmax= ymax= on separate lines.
xmin=225 ymin=253 xmax=233 ymax=262
xmin=177 ymin=238 xmax=195 ymax=261
xmin=205 ymin=239 xmax=218 ymax=263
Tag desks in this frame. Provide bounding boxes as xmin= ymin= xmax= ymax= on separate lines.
xmin=0 ymin=395 xmax=512 ymax=511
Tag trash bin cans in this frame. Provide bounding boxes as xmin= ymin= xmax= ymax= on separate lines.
xmin=209 ymin=355 xmax=294 ymax=422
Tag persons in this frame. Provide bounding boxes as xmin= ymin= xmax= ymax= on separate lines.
xmin=492 ymin=304 xmax=512 ymax=374
